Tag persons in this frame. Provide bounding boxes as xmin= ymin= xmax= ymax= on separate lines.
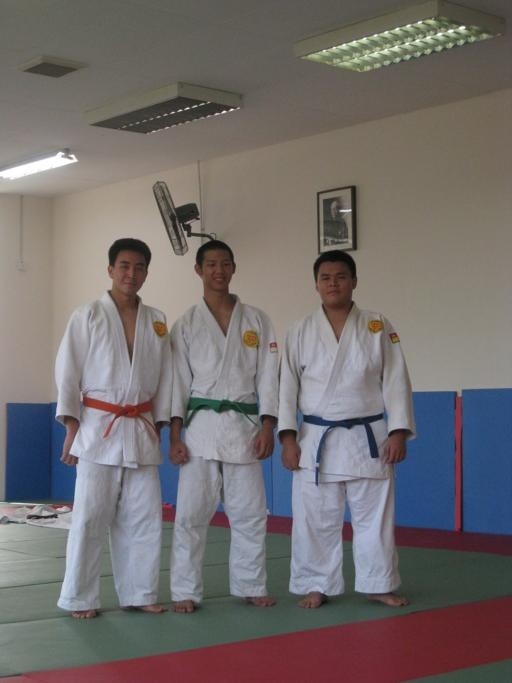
xmin=168 ymin=238 xmax=280 ymax=613
xmin=53 ymin=236 xmax=175 ymax=619
xmin=323 ymin=200 xmax=349 ymax=245
xmin=276 ymin=247 xmax=416 ymax=610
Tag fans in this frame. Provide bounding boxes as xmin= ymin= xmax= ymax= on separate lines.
xmin=153 ymin=181 xmax=214 ymax=255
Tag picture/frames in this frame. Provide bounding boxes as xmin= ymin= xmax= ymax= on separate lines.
xmin=317 ymin=185 xmax=356 ymax=255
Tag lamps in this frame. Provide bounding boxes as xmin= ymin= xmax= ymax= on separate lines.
xmin=293 ymin=1 xmax=506 ymax=72
xmin=0 ymin=147 xmax=78 ymax=181
xmin=85 ymin=82 xmax=243 ymax=134
